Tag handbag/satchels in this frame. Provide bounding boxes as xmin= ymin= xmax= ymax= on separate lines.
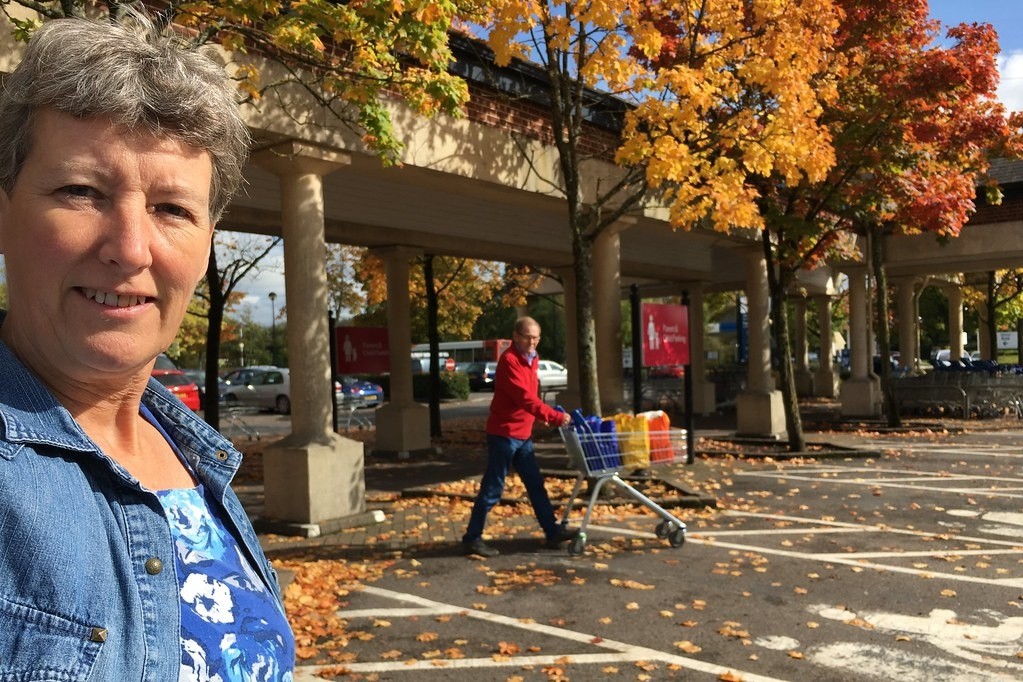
xmin=575 ymin=409 xmax=674 ymax=472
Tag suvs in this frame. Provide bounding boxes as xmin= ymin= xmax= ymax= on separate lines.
xmin=151 ymin=370 xmax=200 ymax=414
xmin=467 ymin=362 xmax=497 ymax=391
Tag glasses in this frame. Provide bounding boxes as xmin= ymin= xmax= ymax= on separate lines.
xmin=517 ymin=331 xmax=541 ymax=342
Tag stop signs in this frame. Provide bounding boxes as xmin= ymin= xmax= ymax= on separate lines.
xmin=446 ymin=359 xmax=455 ymax=371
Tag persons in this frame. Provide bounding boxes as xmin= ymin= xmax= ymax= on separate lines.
xmin=0 ymin=15 xmax=297 ymax=681
xmin=460 ymin=317 xmax=580 ymax=557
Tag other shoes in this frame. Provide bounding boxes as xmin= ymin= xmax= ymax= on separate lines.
xmin=460 ymin=538 xmax=500 ymax=557
xmin=546 ymin=527 xmax=580 ymax=545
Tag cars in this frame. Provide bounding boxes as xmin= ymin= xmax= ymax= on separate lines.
xmin=537 ymin=361 xmax=568 ymax=389
xmin=218 ymin=366 xmax=280 ymax=398
xmin=873 ymin=355 xmax=909 ymax=373
xmin=222 ymin=369 xmax=344 ymax=415
xmin=970 ymin=350 xmax=980 ymax=365
xmin=935 ymin=349 xmax=973 ymax=371
xmin=185 ymin=372 xmax=222 ymax=394
xmin=333 ymin=375 xmax=384 ymax=410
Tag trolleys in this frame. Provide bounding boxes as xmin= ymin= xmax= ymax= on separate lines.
xmin=900 ymin=361 xmax=1023 ymax=420
xmin=553 ymin=404 xmax=690 ymax=558
